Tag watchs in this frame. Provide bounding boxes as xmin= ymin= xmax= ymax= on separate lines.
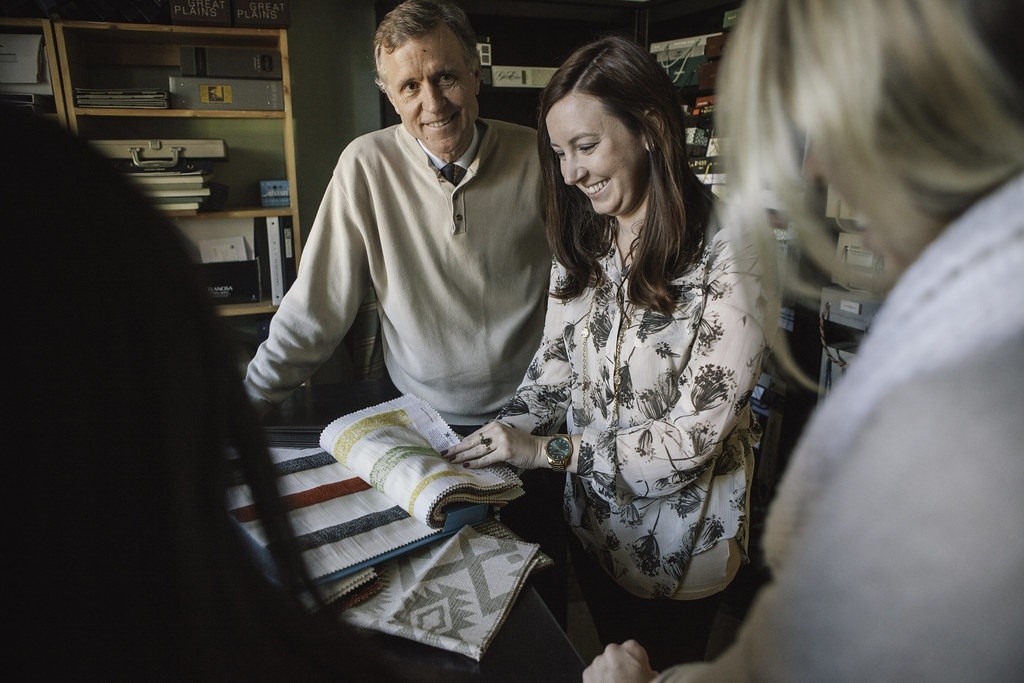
xmin=544 ymin=434 xmax=574 ymax=472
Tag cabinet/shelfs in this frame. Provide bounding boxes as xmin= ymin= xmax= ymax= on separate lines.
xmin=0 ymin=15 xmax=68 ymax=131
xmin=641 ymin=0 xmax=891 ymax=623
xmin=372 ymin=0 xmax=641 ymax=134
xmin=53 ymin=18 xmax=314 ymax=425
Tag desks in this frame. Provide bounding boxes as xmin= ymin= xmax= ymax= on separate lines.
xmin=354 ymin=579 xmax=587 ymax=682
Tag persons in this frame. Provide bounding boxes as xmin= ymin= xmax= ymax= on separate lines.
xmin=585 ymin=0 xmax=1024 ymax=683
xmin=240 ymin=0 xmax=572 ymax=635
xmin=0 ymin=91 xmax=403 ymax=683
xmin=439 ymin=37 xmax=782 ymax=672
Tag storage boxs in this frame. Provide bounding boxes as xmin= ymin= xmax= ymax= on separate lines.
xmin=650 ymin=8 xmax=888 ymax=512
xmin=173 ymin=217 xmax=260 ymax=303
xmin=231 ymin=0 xmax=290 ymax=28
xmin=170 ymin=0 xmax=231 ymax=26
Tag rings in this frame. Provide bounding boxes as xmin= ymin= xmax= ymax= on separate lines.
xmin=479 ymin=433 xmax=493 ymax=452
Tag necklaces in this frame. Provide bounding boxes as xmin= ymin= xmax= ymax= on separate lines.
xmin=581 ymin=267 xmax=637 ymax=425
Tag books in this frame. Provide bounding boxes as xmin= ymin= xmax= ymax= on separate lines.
xmin=121 ymin=170 xmax=212 ymax=217
